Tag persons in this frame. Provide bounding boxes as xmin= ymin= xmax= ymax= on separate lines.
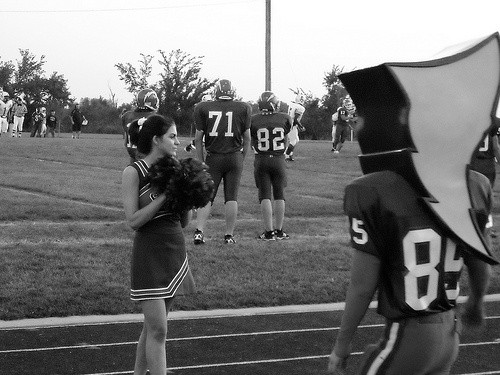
xmin=121 ymin=115 xmax=196 ymax=375
xmin=284 ymin=95 xmax=306 ymax=162
xmin=250 ymin=91 xmax=293 ymax=241
xmin=125 ymin=80 xmax=253 ymax=245
xmin=327 ymin=30 xmax=500 ymax=375
xmin=0 ymin=92 xmax=57 ymax=138
xmin=69 ymin=103 xmax=87 ymax=139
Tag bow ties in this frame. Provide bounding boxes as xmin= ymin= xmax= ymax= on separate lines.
xmin=17 ymin=104 xmax=22 ymax=106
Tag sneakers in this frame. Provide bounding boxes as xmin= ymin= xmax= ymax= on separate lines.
xmin=274 ymin=229 xmax=290 ymax=240
xmin=194 ymin=229 xmax=205 ymax=244
xmin=256 ymin=230 xmax=275 ymax=240
xmin=224 ymin=234 xmax=236 ymax=244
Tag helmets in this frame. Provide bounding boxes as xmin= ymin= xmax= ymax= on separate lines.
xmin=214 ymin=80 xmax=234 ymax=100
xmin=257 ymin=92 xmax=279 ymax=111
xmin=137 ymin=88 xmax=160 ymax=112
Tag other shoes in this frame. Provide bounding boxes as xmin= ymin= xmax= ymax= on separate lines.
xmin=285 ymin=154 xmax=295 ymax=161
